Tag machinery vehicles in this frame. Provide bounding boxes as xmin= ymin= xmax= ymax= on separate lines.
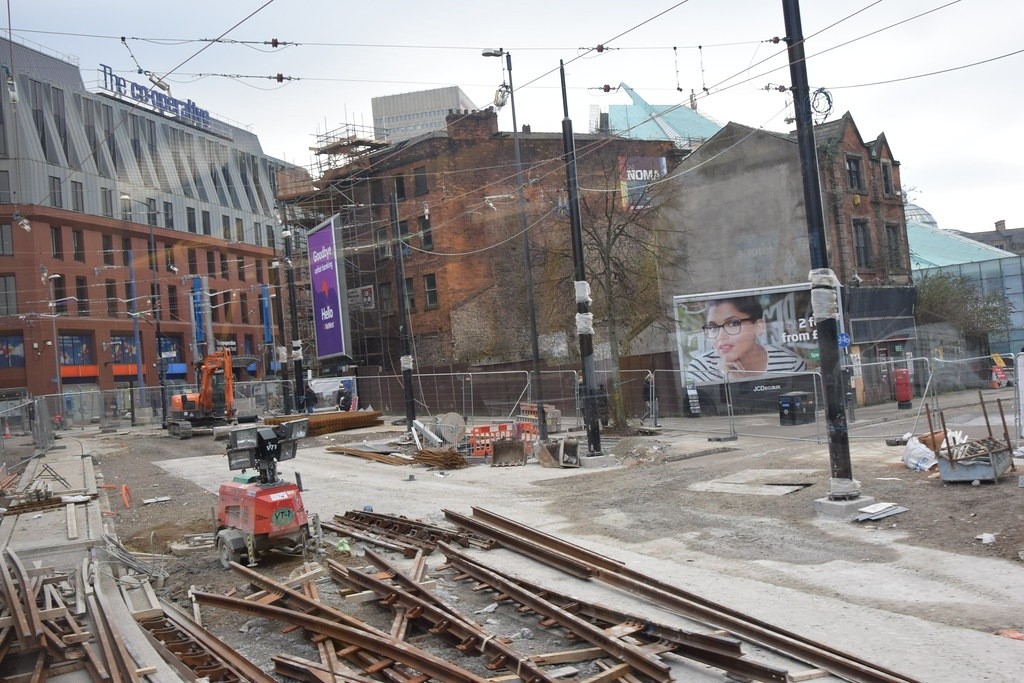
xmin=209 ymin=418 xmax=323 ymax=571
xmin=166 ymin=344 xmax=258 ymax=437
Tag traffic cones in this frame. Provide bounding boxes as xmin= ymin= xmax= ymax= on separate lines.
xmin=4 ymin=423 xmax=13 ymax=439
xmin=990 ymin=370 xmax=1002 ymax=390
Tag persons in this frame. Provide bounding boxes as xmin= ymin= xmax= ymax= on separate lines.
xmin=340 ymin=389 xmax=351 ymax=411
xmin=304 ymin=385 xmax=317 ymax=413
xmin=597 ymin=383 xmax=609 ymax=429
xmin=687 ymin=296 xmax=809 ymax=384
xmin=639 ymin=373 xmax=662 ymax=427
xmin=337 ymin=383 xmax=346 ymax=406
xmin=25 ymin=400 xmax=35 ymax=432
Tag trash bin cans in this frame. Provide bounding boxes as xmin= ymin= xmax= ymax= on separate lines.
xmin=777 ymin=390 xmax=816 ymax=426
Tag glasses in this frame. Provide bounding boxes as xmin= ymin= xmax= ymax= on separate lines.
xmin=701 ymin=317 xmax=751 ymax=339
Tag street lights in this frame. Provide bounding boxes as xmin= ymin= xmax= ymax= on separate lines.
xmin=120 ymin=194 xmax=167 ymax=428
xmin=481 ymin=48 xmax=550 ymax=445
xmin=49 ymin=273 xmax=68 ymax=428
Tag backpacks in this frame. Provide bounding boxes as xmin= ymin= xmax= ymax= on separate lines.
xmin=339 ymin=390 xmax=352 ymax=407
xmin=306 ymin=390 xmax=317 ymax=406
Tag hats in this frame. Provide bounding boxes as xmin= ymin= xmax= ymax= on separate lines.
xmin=339 ymin=384 xmax=343 ymax=388
